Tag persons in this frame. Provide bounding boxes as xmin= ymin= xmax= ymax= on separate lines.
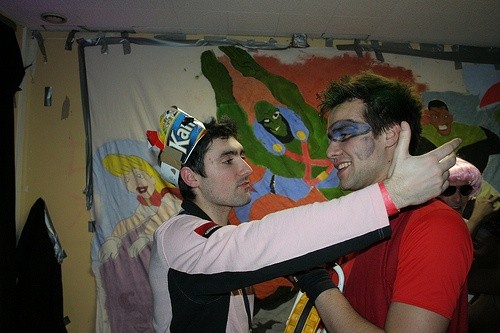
xmin=147 ymin=105 xmax=462 ymax=333
xmin=285 ymin=72 xmax=500 ymax=333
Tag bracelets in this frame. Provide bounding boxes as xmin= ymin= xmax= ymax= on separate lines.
xmin=377 ymin=183 xmax=402 ymax=217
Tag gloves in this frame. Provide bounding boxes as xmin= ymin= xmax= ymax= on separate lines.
xmin=292 ymin=265 xmax=340 ymax=306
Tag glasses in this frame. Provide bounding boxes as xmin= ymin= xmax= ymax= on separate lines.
xmin=441 ymin=185 xmax=474 ymax=196
